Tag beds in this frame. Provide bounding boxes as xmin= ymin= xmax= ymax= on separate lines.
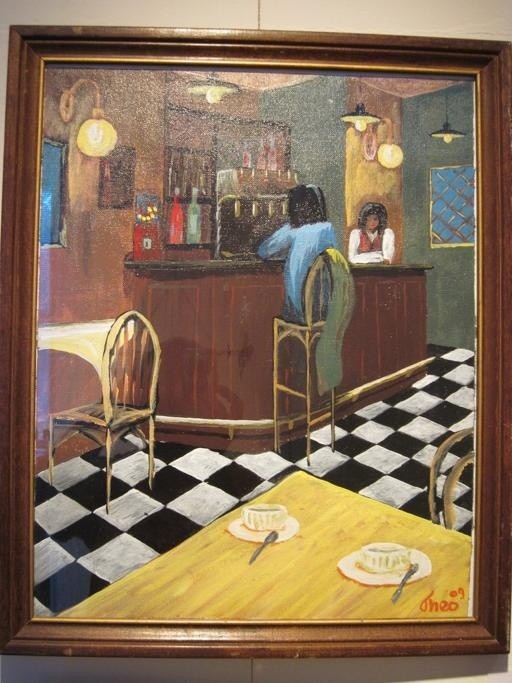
xmin=57 ymin=471 xmax=474 ymax=622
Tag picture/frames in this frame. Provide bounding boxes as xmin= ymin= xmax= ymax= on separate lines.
xmin=2 ymin=25 xmax=512 ymax=660
xmin=98 ymin=145 xmax=137 ymax=210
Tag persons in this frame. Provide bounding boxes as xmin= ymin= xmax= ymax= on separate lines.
xmin=255 ymin=182 xmax=341 ymax=327
xmin=347 ymin=200 xmax=397 ymax=266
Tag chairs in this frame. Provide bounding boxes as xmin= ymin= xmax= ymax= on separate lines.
xmin=270 ymin=247 xmax=355 ymax=469
xmin=429 ymin=428 xmax=474 ymax=533
xmin=46 ymin=308 xmax=163 ymax=512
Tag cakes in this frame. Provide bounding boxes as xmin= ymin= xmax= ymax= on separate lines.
xmin=242 ymin=504 xmax=288 ymax=530
xmin=359 ymin=543 xmax=408 ymax=574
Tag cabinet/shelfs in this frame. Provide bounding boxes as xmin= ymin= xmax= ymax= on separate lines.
xmin=163 ymin=105 xmax=292 ymax=260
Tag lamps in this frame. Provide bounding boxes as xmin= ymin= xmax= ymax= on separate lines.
xmin=342 ymin=81 xmax=380 ymax=131
xmin=361 ymin=117 xmax=404 ymax=170
xmin=429 ymin=83 xmax=464 ymax=143
xmin=187 ymin=69 xmax=241 ymax=104
xmin=59 ymin=77 xmax=119 ymax=158
